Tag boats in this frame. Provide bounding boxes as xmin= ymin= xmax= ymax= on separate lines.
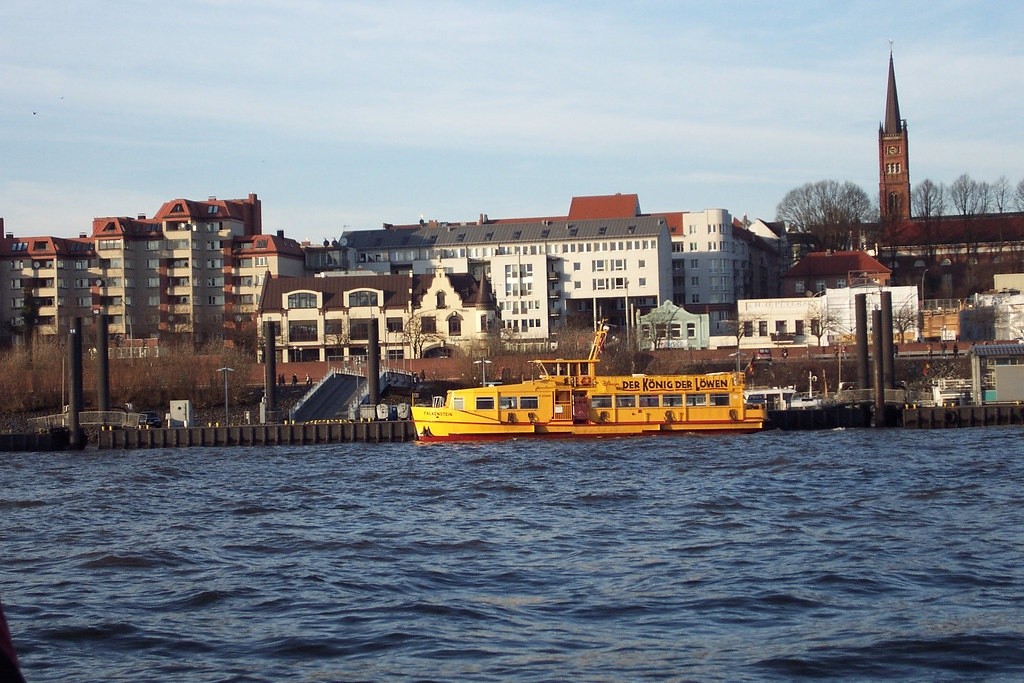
xmin=742 ymin=387 xmax=826 ymax=407
xmin=410 ymin=316 xmax=768 ymax=444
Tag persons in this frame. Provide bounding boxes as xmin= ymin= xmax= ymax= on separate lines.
xmin=305 ymin=374 xmax=312 ymax=385
xmin=278 ymin=373 xmax=285 ymax=385
xmin=834 ymin=344 xmax=847 ymax=360
xmin=413 ymin=370 xmax=425 ymax=384
xmin=941 ymin=340 xmax=947 ymax=356
xmin=983 ymin=339 xmax=988 ymax=345
xmin=781 ymin=347 xmax=788 ymax=359
xmin=893 ymin=343 xmax=898 ymax=358
xmin=953 ymin=341 xmax=959 ymax=358
xmin=291 ymin=373 xmax=298 ymax=385
xmin=993 ymin=338 xmax=998 ymax=345
xmin=926 ymin=343 xmax=933 ymax=358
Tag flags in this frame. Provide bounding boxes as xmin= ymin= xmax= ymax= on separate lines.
xmin=743 ymin=356 xmax=754 ymax=376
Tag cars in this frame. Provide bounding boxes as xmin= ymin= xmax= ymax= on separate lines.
xmin=139 ymin=411 xmax=164 ymax=429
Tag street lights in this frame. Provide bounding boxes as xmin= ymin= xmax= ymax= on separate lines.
xmin=922 ymin=267 xmax=929 ymax=310
xmin=216 ymin=367 xmax=236 ymax=425
xmin=59 ymin=342 xmax=67 ymax=426
xmin=474 ymin=359 xmax=492 ymax=385
